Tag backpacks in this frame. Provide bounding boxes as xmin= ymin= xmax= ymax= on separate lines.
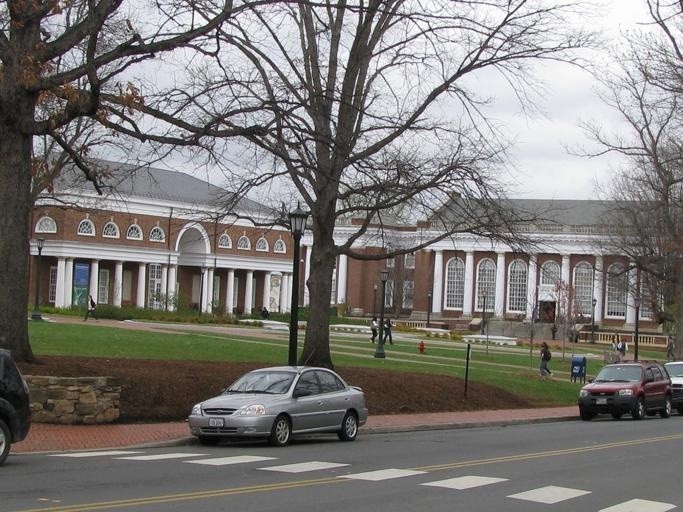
xmin=544 ymin=349 xmax=551 ymax=361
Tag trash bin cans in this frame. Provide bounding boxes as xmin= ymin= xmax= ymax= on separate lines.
xmin=571 ymin=356 xmax=586 ymax=384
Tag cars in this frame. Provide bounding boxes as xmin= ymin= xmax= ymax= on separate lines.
xmin=645 ymin=361 xmax=683 ymax=417
xmin=468 ymin=318 xmax=482 ymax=331
xmin=576 ymin=360 xmax=673 ymax=421
xmin=187 ymin=366 xmax=368 ymax=448
xmin=0 ymin=348 xmax=31 ymax=463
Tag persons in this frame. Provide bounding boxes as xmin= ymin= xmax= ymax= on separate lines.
xmin=537 ymin=344 xmax=547 ymax=377
xmin=665 ymin=336 xmax=674 ymax=358
xmin=616 ymin=337 xmax=628 ymax=361
xmin=382 ymin=318 xmax=394 ymax=346
xmin=82 ymin=294 xmax=98 ymax=323
xmin=541 ymin=341 xmax=553 ymax=376
xmin=345 ymin=302 xmax=351 ymax=318
xmin=549 ymin=323 xmax=557 ymax=341
xmin=260 ymin=306 xmax=270 ymax=321
xmin=369 ymin=317 xmax=379 ymax=344
xmin=610 ymin=337 xmax=617 ymax=362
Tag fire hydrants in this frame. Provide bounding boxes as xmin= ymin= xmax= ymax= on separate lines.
xmin=417 ymin=341 xmax=425 ymax=352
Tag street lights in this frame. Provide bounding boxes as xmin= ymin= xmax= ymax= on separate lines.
xmin=31 ymin=236 xmax=45 ymax=319
xmin=288 ymin=200 xmax=308 ymax=367
xmin=427 ymin=290 xmax=432 ymax=327
xmin=591 ymin=298 xmax=596 ymax=344
xmin=633 ymin=298 xmax=640 ymax=360
xmin=481 ymin=287 xmax=487 ymax=334
xmin=372 ymin=285 xmax=377 ymax=318
xmin=374 ymin=268 xmax=389 ymax=358
